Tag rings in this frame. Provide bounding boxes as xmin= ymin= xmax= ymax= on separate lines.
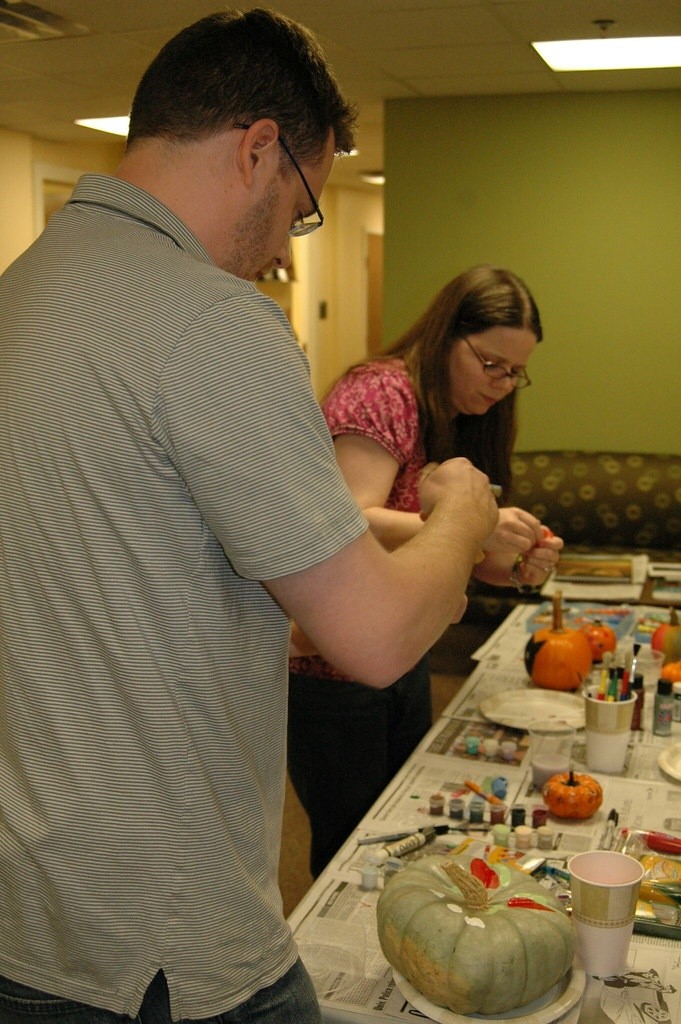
xmin=545 ymin=560 xmax=553 ymax=572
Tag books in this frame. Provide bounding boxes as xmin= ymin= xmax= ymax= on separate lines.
xmin=555 ymin=556 xmax=633 ymax=583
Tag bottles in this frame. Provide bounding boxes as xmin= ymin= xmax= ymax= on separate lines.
xmin=653 ymin=679 xmax=672 ymax=736
xmin=631 ymin=673 xmax=644 ymax=730
xmin=671 ymin=682 xmax=681 ymax=721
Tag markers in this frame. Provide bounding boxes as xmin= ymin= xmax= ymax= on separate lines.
xmin=463 ymin=778 xmax=509 ymax=809
xmin=357 ymin=824 xmax=450 ymax=845
xmin=600 ymin=809 xmax=619 ymax=851
xmin=374 ymin=828 xmax=437 ymax=865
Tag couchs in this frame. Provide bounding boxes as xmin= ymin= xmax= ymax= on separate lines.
xmin=427 ymin=449 xmax=681 ymax=676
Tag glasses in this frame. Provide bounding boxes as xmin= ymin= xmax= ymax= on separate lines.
xmin=234 ymin=123 xmax=324 ymax=238
xmin=456 ymin=326 xmax=532 ymax=389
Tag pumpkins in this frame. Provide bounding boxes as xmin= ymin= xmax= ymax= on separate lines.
xmin=524 ymin=590 xmax=593 ymax=692
xmin=651 ymin=607 xmax=681 ymax=683
xmin=579 ymin=619 xmax=615 ymax=663
xmin=542 ymin=769 xmax=603 ymax=820
xmin=375 ymin=854 xmax=578 ymax=1016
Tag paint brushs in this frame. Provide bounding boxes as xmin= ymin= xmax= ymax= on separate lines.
xmin=578 ymin=643 xmax=641 ymax=701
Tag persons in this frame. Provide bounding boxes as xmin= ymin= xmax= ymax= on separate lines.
xmin=0 ymin=9 xmax=499 ymax=1024
xmin=289 ymin=264 xmax=565 ymax=881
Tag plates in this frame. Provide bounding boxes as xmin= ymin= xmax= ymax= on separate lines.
xmin=480 ymin=688 xmax=587 ymax=730
xmin=657 ymin=742 xmax=681 ymax=781
xmin=392 ymin=953 xmax=586 ymax=1024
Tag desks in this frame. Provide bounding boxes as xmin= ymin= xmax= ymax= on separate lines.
xmin=288 ymin=603 xmax=681 ymax=1024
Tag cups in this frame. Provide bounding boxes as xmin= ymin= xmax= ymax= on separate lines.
xmin=581 ymin=685 xmax=638 ymax=775
xmin=527 ymin=721 xmax=577 ymax=788
xmin=625 ymin=649 xmax=665 ymax=697
xmin=567 ymin=850 xmax=645 ymax=973
